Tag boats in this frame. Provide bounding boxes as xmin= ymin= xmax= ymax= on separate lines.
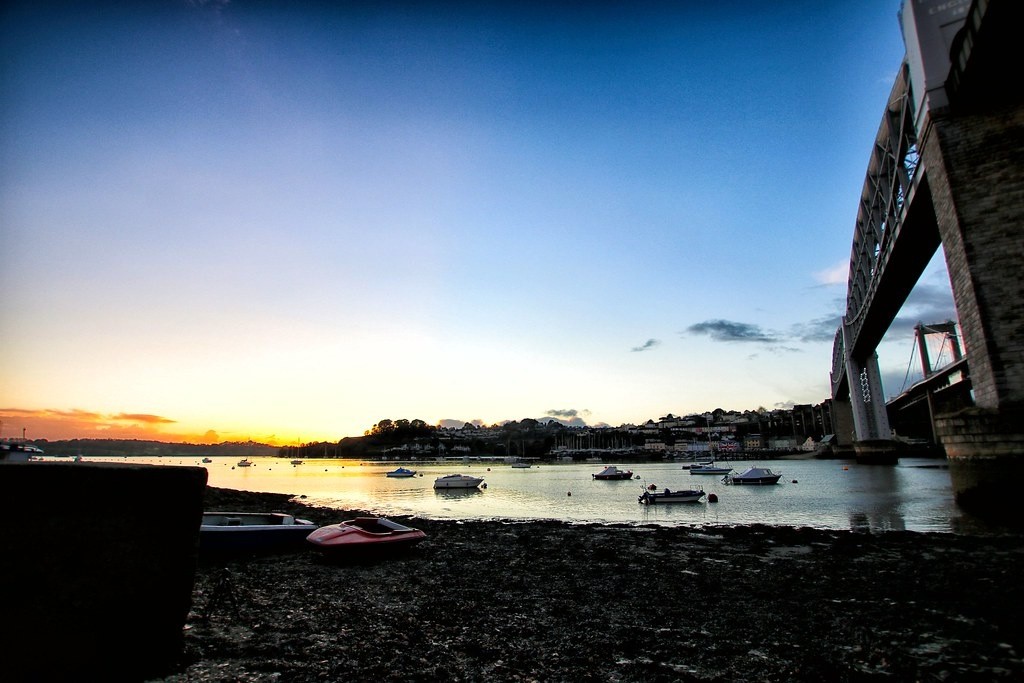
xmin=433 ymin=473 xmax=484 ymax=489
xmin=586 ymin=457 xmax=604 ymax=464
xmin=202 ymin=457 xmax=213 ymax=463
xmin=238 ymin=462 xmax=252 ymax=466
xmin=721 ymin=465 xmax=782 ymax=486
xmin=511 ymin=457 xmax=532 ymax=468
xmin=305 ymin=513 xmax=428 ymax=560
xmin=386 ymin=466 xmax=417 ymax=478
xmin=290 ymin=460 xmax=302 ymax=464
xmin=197 ymin=510 xmax=318 ymax=552
xmin=637 ymin=479 xmax=708 ymax=505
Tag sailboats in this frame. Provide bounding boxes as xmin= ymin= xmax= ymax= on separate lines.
xmin=689 ymin=420 xmax=733 ymax=475
xmin=682 ymin=437 xmax=714 ymax=470
xmin=592 ymin=466 xmax=633 ymax=481
